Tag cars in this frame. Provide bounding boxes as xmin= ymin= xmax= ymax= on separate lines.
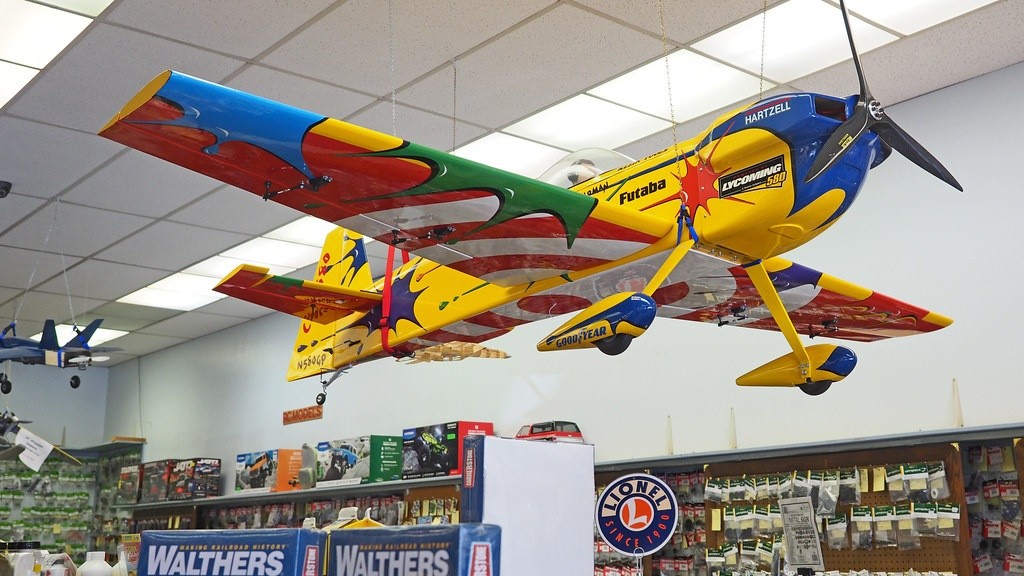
xmin=416 ymin=432 xmax=454 ymax=475
xmin=249 ymin=453 xmax=274 ymax=479
xmin=332 ymin=443 xmax=358 ymax=469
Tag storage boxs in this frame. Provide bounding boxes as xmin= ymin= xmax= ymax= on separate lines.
xmin=402 ymin=421 xmax=493 ymax=479
xmin=460 ymin=435 xmax=595 ymax=576
xmin=235 ymin=449 xmax=304 ymax=492
xmin=117 ymin=534 xmax=142 ymax=576
xmin=115 ymin=458 xmax=221 ymax=505
xmin=317 ymin=435 xmax=403 ymax=484
xmin=137 ymin=528 xmax=328 ymax=576
xmin=327 ymin=522 xmax=501 ymax=576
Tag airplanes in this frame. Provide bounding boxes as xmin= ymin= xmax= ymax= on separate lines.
xmin=96 ymin=2 xmax=963 ymax=406
xmin=0 ymin=318 xmax=105 ymax=394
xmin=0 ymin=406 xmax=85 ymax=468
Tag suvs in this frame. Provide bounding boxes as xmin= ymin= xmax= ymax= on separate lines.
xmin=514 ymin=420 xmax=585 ymax=444
